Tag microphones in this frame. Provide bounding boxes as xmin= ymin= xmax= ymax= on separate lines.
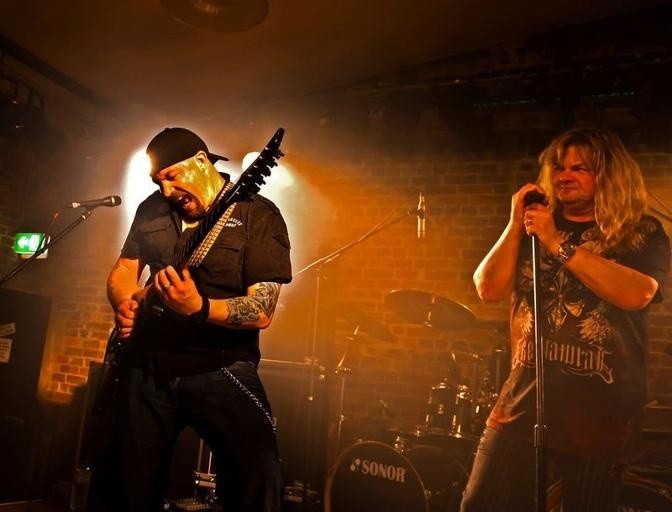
xmin=524 ymin=190 xmax=547 ymax=206
xmin=64 ymin=195 xmax=122 ymax=210
xmin=415 ymin=193 xmax=427 ymax=240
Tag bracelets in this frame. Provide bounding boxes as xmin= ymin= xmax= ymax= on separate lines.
xmin=192 ymin=294 xmax=213 ymax=324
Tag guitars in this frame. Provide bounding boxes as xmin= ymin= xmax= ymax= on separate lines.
xmin=88 ymin=126 xmax=287 ymax=445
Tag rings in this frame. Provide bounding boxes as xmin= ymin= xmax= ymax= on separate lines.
xmin=528 ymin=217 xmax=533 ymax=226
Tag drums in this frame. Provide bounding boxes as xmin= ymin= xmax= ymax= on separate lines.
xmin=426 ymin=379 xmax=469 ymax=435
xmin=395 ymin=426 xmax=466 ymax=512
xmin=324 ymin=439 xmax=430 ymax=512
xmin=453 ymin=390 xmax=500 ymax=443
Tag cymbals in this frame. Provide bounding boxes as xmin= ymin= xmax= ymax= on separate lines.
xmin=435 ymin=350 xmax=484 ymax=365
xmin=384 ymin=289 xmax=478 ymax=332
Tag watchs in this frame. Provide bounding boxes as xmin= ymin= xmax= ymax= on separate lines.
xmin=551 ymin=238 xmax=576 ymax=268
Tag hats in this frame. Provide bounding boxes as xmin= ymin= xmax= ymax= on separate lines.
xmin=147 ymin=127 xmax=230 ymax=178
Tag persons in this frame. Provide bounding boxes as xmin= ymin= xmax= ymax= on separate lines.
xmin=456 ymin=117 xmax=672 ymax=512
xmin=105 ymin=126 xmax=294 ymax=510
